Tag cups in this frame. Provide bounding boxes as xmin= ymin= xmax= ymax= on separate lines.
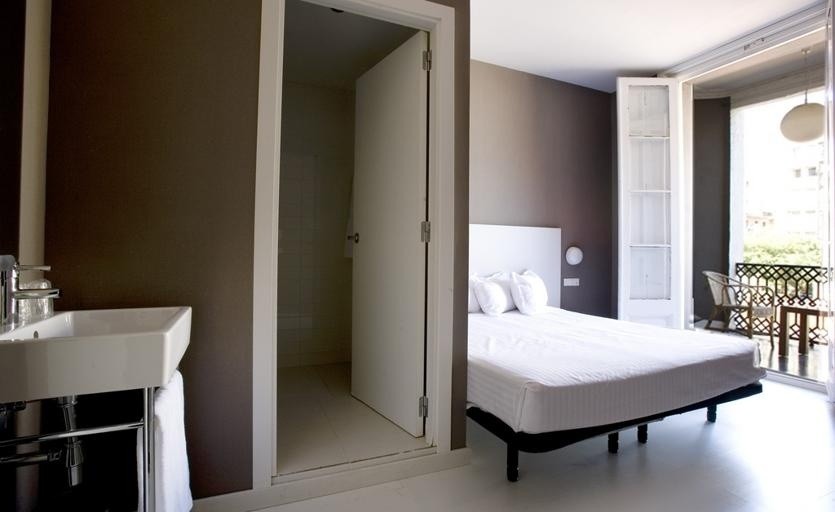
xmin=27 ymin=279 xmax=54 ymax=318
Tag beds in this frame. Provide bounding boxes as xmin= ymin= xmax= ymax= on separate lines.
xmin=466 ymin=303 xmax=766 ymax=483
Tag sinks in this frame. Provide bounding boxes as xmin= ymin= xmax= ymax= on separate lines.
xmin=0 ymin=307 xmax=193 ymax=403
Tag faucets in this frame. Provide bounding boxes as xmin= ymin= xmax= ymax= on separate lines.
xmin=3 ymin=261 xmax=59 ymax=333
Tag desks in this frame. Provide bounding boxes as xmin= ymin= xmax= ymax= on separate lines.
xmin=778 ymin=304 xmax=833 ymax=357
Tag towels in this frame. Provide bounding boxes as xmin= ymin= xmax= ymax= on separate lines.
xmin=133 ymin=371 xmax=196 ymax=512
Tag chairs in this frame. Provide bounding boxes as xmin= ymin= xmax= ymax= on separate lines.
xmin=702 ymin=269 xmax=775 ymax=350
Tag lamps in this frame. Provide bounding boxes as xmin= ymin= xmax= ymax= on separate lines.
xmin=565 ymin=246 xmax=584 ymax=267
xmin=780 ymin=47 xmax=825 ymax=143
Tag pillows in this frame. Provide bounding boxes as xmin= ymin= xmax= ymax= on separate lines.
xmin=467 ymin=269 xmax=548 ymax=317
